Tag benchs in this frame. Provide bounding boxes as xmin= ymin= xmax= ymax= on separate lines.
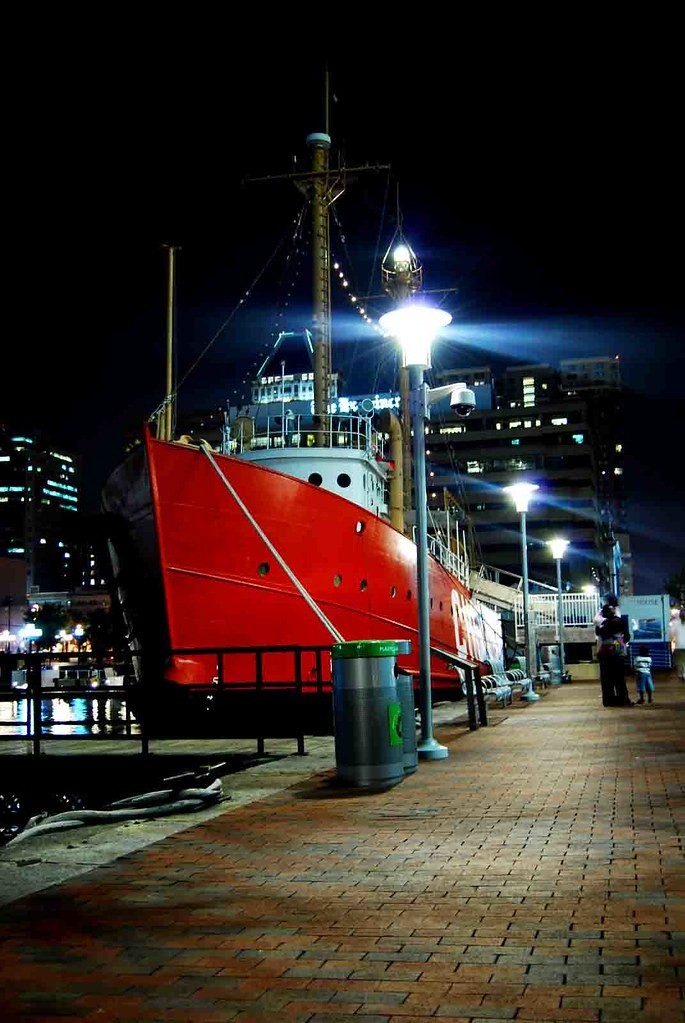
xmin=448 ymin=656 xmax=561 ymax=706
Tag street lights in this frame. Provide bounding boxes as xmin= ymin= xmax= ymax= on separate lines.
xmin=377 ymin=299 xmax=453 ymax=760
xmin=545 ymin=536 xmax=571 ymax=678
xmin=54 ymin=630 xmax=73 ymax=653
xmin=502 ymin=482 xmax=540 ymax=702
xmin=19 ymin=624 xmax=42 ymax=654
xmin=0 ymin=629 xmax=16 ymax=654
xmin=75 ymin=624 xmax=84 ymax=665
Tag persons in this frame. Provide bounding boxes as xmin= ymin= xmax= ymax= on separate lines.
xmin=285 ymin=409 xmax=296 ymax=447
xmin=311 ymin=415 xmax=326 ymax=446
xmin=672 ymin=609 xmax=685 ymax=678
xmin=633 ymin=643 xmax=655 ymax=705
xmin=592 ymin=593 xmax=636 ymax=707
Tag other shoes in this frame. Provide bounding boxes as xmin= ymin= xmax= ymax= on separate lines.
xmin=604 ymin=695 xmax=617 ymax=707
xmin=617 ymin=699 xmax=635 ymax=707
xmin=648 ymin=698 xmax=652 ymax=703
xmin=638 ymin=699 xmax=644 ymax=704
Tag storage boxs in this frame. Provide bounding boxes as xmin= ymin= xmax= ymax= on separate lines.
xmin=628 ymin=641 xmax=672 ymax=668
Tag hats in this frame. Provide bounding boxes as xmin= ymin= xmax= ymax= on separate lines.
xmin=601 ymin=604 xmax=615 ymax=613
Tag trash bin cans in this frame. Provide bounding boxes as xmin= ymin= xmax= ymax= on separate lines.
xmin=329 ymin=639 xmax=419 ymax=791
xmin=541 ymin=645 xmax=561 ymax=685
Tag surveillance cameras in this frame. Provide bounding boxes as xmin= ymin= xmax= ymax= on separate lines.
xmin=450 ymin=389 xmax=476 ymax=417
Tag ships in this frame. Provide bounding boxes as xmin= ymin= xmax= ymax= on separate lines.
xmin=93 ymin=66 xmax=504 ymax=739
